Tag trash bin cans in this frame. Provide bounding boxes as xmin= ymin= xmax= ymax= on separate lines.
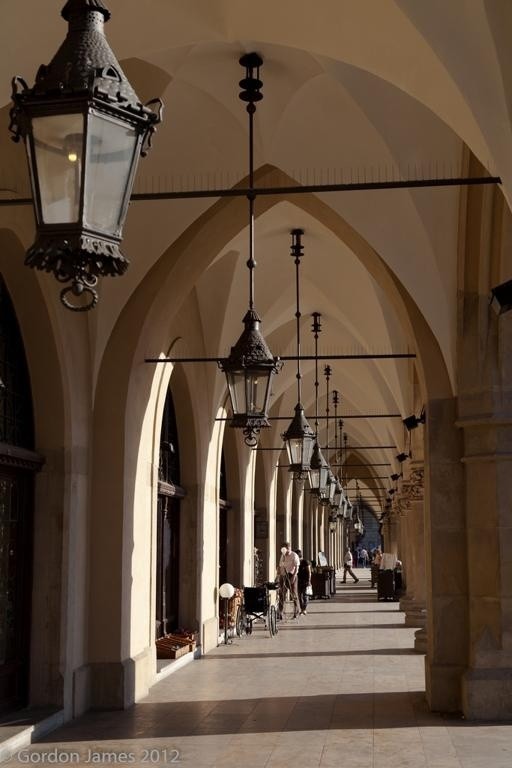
xmin=377 ymin=568 xmax=402 ymax=602
xmin=311 ymin=565 xmax=335 ymax=600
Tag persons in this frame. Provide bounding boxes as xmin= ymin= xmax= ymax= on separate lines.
xmin=352 ymin=545 xmax=381 ymax=567
xmin=293 ymin=549 xmax=312 ymax=615
xmin=274 ymin=542 xmax=302 ymax=621
xmin=340 ymin=546 xmax=360 ymax=583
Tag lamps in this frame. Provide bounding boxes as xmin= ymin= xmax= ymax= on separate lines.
xmin=380 ymin=411 xmax=427 ymax=522
xmin=304 ymin=312 xmax=367 ymax=527
xmin=10 ymin=0 xmax=168 ymax=311
xmin=282 ymin=229 xmax=319 ymax=481
xmin=489 ymin=277 xmax=511 ymax=314
xmin=221 ymin=53 xmax=281 ymax=450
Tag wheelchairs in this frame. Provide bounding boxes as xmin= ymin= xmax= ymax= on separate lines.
xmin=236 ymin=584 xmax=280 ymax=639
